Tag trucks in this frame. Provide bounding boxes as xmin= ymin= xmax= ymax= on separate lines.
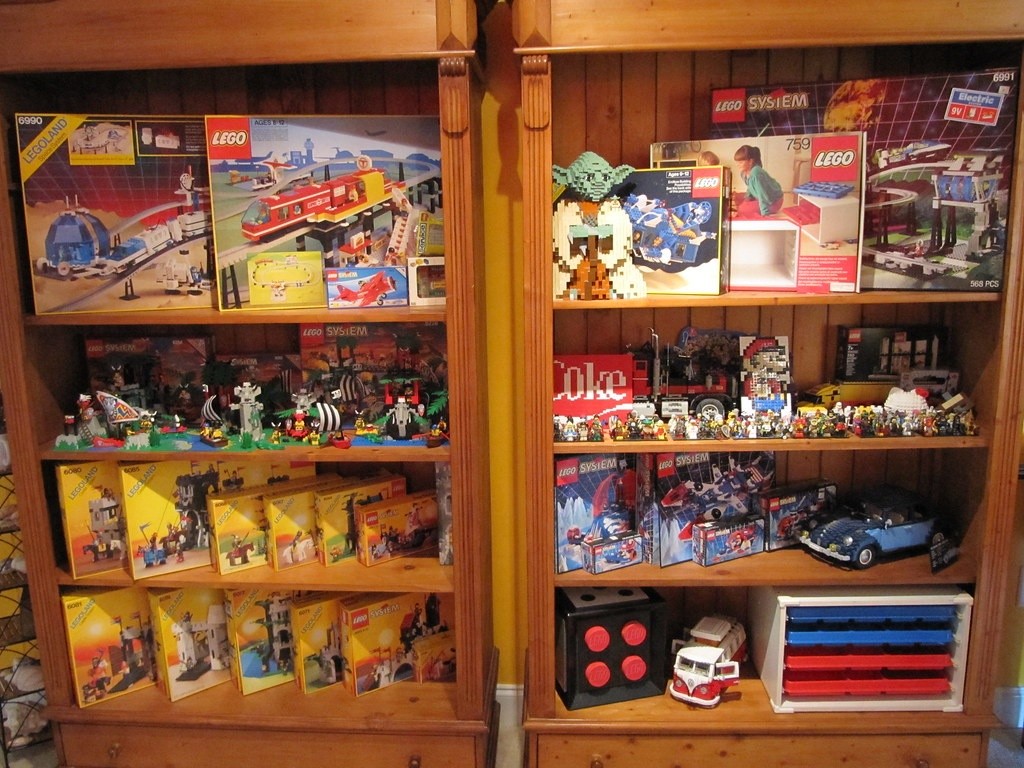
xmin=663 ymin=612 xmax=751 ymax=709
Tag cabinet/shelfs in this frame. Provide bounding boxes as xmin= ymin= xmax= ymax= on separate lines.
xmin=0 ymin=0 xmax=1024 ymax=768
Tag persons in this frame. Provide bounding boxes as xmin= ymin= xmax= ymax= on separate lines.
xmin=734 ymin=145 xmax=784 ymax=218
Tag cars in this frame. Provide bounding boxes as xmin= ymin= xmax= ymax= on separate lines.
xmin=799 ymin=482 xmax=958 ymax=571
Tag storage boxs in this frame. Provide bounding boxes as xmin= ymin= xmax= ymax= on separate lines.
xmin=55 ymin=460 xmax=133 ymax=580
xmin=145 ymin=588 xmax=231 ymax=702
xmin=408 ymin=257 xmax=447 ymax=307
xmin=116 ymin=461 xmax=317 ymax=579
xmin=262 ymin=476 xmax=366 ymax=573
xmin=205 ymin=476 xmax=340 ymax=576
xmin=553 ymin=69 xmax=1019 ymax=292
xmin=84 ymin=323 xmax=449 ymax=411
xmin=61 ymin=587 xmax=157 ymax=707
xmin=314 ymin=474 xmax=406 ymax=568
xmin=555 ymin=455 xmax=837 ymax=575
xmin=16 ymin=113 xmax=219 ymax=316
xmin=287 ymin=593 xmax=341 ymax=695
xmin=337 ymin=592 xmax=456 ymax=699
xmin=413 ymin=630 xmax=456 ymax=685
xmin=204 ymin=113 xmax=441 ymax=309
xmin=354 ymin=490 xmax=439 ymax=567
xmin=327 ymin=265 xmax=409 ymax=309
xmin=553 ymin=324 xmax=956 ymax=430
xmin=222 ymin=587 xmax=327 ymax=696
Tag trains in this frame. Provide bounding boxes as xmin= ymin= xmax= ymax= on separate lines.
xmin=239 ymin=163 xmax=408 ymax=239
xmin=26 ymin=194 xmax=213 ymax=280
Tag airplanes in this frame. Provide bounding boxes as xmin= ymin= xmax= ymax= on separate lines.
xmin=330 ymin=266 xmax=398 ymax=309
